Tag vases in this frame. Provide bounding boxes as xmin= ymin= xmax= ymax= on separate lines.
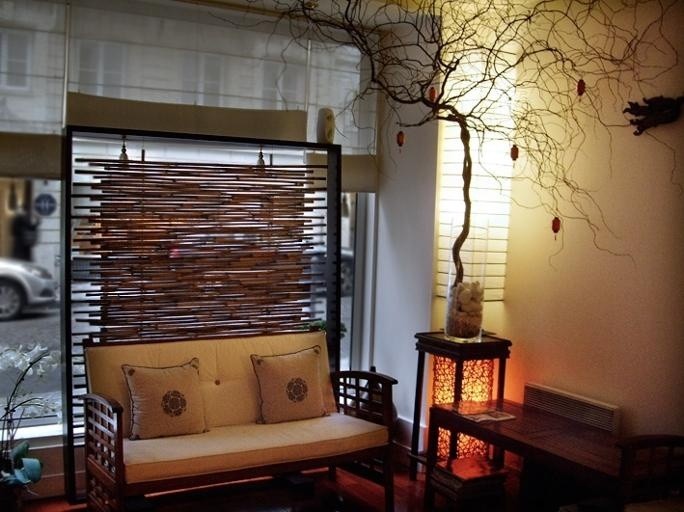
xmin=445 ymin=214 xmax=483 ymax=343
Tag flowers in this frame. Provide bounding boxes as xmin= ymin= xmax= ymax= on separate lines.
xmin=0 ymin=343 xmax=63 ymax=474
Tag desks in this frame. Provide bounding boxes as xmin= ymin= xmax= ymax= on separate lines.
xmin=418 ymin=397 xmax=616 ymax=511
xmin=408 ymin=329 xmax=513 ymax=486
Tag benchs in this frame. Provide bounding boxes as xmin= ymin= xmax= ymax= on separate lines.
xmin=81 ymin=327 xmax=397 ymax=511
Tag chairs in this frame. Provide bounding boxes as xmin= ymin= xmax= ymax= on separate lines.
xmin=615 ymin=433 xmax=684 ymax=512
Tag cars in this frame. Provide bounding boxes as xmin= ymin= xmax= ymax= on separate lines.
xmin=303 ymin=244 xmax=354 ymax=297
xmin=0 ymin=255 xmax=58 ymax=321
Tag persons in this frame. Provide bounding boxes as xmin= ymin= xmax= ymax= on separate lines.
xmin=6 ymin=202 xmax=39 ymax=264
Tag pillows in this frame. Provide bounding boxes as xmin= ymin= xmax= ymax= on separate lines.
xmin=119 ymin=343 xmax=330 ymax=441
